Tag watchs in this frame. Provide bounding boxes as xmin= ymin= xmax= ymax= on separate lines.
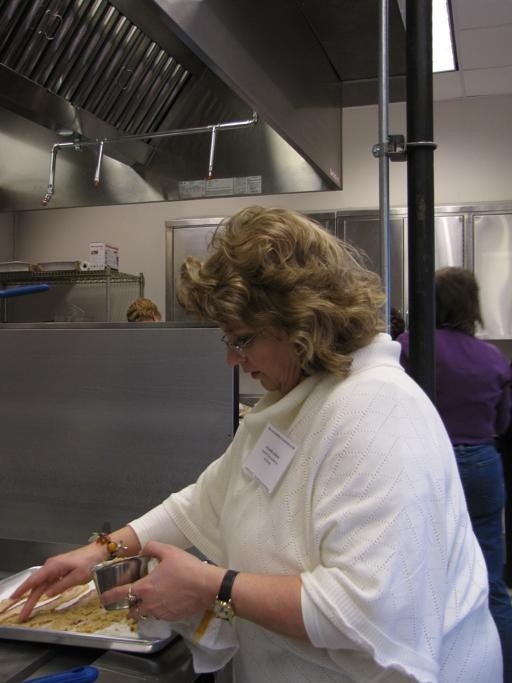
xmin=212 ymin=569 xmax=238 ymax=619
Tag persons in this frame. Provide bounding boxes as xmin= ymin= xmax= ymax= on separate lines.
xmin=126 ymin=299 xmax=162 ymax=322
xmin=9 ymin=203 xmax=506 ymax=682
xmin=394 ymin=266 xmax=512 ymax=683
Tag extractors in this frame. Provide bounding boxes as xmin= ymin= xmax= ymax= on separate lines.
xmin=1 ymin=2 xmax=342 ymax=213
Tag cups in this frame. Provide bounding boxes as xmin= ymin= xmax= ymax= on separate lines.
xmin=89 ymin=555 xmax=151 ymax=611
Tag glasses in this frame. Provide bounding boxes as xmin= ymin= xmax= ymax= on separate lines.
xmin=220 ymin=328 xmax=267 ymax=353
